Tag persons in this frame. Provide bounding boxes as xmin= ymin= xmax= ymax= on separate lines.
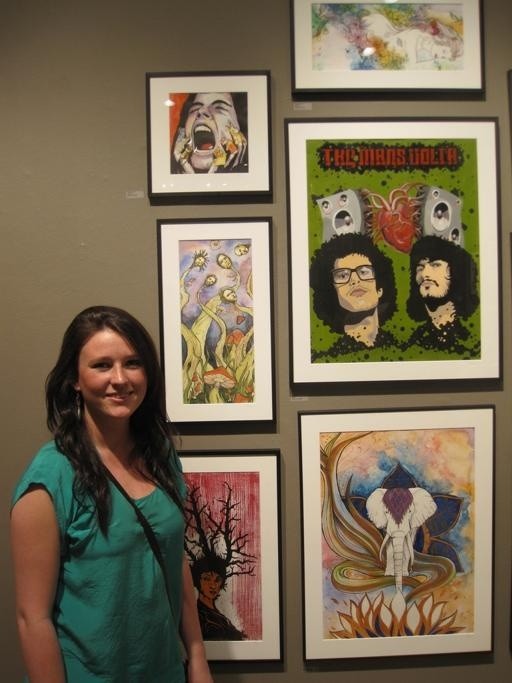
xmin=310 ymin=233 xmax=482 ymax=363
xmin=169 ymin=93 xmax=248 ymax=173
xmin=0 ymin=305 xmax=214 ymax=683
xmin=189 ymin=554 xmax=247 ymax=640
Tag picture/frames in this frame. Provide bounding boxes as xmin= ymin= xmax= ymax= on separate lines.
xmin=155 ymin=216 xmax=277 ymax=427
xmin=284 ymin=117 xmax=505 ymax=395
xmin=175 ymin=448 xmax=284 ymax=669
xmin=290 ymin=0 xmax=486 ymax=101
xmin=144 ymin=71 xmax=272 ymax=201
xmin=297 ymin=406 xmax=492 ymax=670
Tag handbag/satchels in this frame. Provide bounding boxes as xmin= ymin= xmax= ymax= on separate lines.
xmin=176 ymin=632 xmax=189 ymax=666
